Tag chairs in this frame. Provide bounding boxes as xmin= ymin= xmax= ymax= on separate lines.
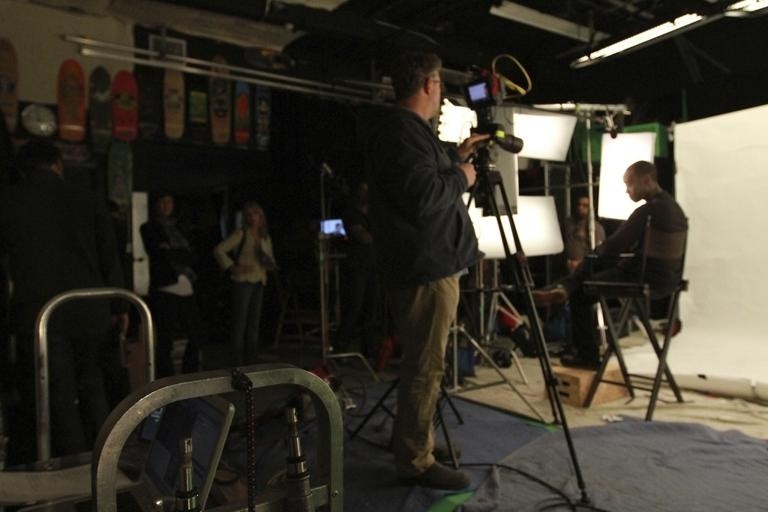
xmin=580 ymin=212 xmax=689 ymax=424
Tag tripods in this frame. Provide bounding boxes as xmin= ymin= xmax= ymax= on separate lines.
xmin=349 ymin=372 xmax=467 ymax=470
xmin=484 ymin=260 xmax=531 ymax=346
xmin=437 ymin=314 xmax=551 ymax=427
xmin=465 ymin=259 xmax=532 ymax=388
xmin=305 ymin=232 xmax=384 ymax=384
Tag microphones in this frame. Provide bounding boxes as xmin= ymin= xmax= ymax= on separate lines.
xmin=322 ymin=162 xmax=334 ymax=177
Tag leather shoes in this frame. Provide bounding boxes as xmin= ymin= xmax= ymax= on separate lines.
xmin=532 ymin=282 xmax=568 ymax=305
xmin=395 ymin=446 xmax=471 ymax=489
xmin=562 ymin=354 xmax=603 ymax=370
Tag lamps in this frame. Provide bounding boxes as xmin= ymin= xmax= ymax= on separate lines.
xmin=486 ymin=0 xmax=767 ymax=71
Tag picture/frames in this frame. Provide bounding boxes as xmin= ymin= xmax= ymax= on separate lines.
xmin=146 ymin=34 xmax=188 ymax=67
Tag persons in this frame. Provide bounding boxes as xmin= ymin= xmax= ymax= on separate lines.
xmin=372 ymin=53 xmax=491 ymax=490
xmin=213 ymin=201 xmax=276 ymax=363
xmin=139 ymin=189 xmax=203 ymax=377
xmin=336 ymin=181 xmax=394 ymax=364
xmin=294 ymin=221 xmax=346 ymax=343
xmin=558 ymin=196 xmax=606 ymax=356
xmin=1 ymin=138 xmax=130 ymax=470
xmin=530 ymin=160 xmax=688 ymax=368
